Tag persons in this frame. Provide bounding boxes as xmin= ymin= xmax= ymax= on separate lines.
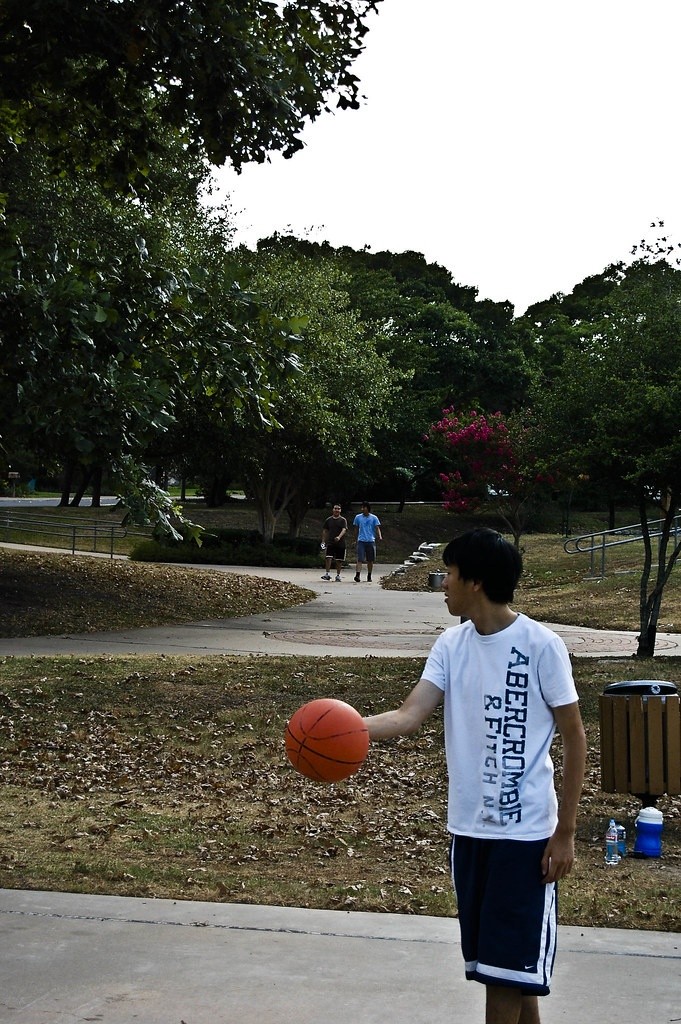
xmin=353 ymin=500 xmax=382 ymax=583
xmin=363 ymin=527 xmax=587 ymax=1024
xmin=321 ymin=504 xmax=348 ymax=582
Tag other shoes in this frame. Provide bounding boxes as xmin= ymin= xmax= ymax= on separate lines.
xmin=367 ymin=573 xmax=372 ymax=582
xmin=354 ymin=572 xmax=360 ymax=582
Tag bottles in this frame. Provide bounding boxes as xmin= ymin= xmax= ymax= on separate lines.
xmin=604 ymin=820 xmax=619 ymax=866
xmin=613 ymin=820 xmax=629 ymax=858
xmin=634 ymin=807 xmax=664 ymax=858
xmin=321 ymin=544 xmax=326 ymax=549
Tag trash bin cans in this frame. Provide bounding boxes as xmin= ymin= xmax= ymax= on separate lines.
xmin=597 ymin=680 xmax=681 ymax=810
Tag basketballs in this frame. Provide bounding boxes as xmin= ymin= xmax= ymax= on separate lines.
xmin=284 ymin=697 xmax=371 ymax=784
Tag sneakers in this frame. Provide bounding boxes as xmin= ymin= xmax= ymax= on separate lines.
xmin=335 ymin=575 xmax=341 ymax=581
xmin=321 ymin=575 xmax=331 ymax=580
